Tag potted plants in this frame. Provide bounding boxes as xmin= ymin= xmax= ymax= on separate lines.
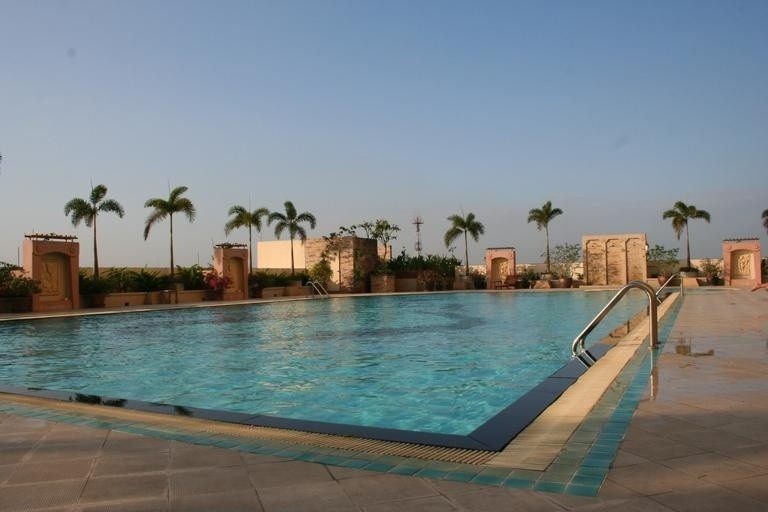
xmin=540 ymin=240 xmax=582 ymax=287
xmin=700 ymin=257 xmax=724 ymax=285
xmin=647 ymin=245 xmax=679 ymax=287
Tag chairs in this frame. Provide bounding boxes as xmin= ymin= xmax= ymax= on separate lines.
xmin=496 ymin=281 xmax=508 ymax=290
